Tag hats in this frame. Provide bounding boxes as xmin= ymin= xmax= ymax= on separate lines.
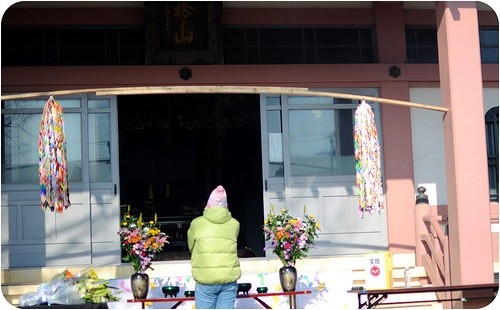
xmin=204 ymin=185 xmax=228 ymax=211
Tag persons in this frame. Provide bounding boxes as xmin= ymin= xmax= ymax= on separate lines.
xmin=186 ymin=185 xmax=242 ymax=309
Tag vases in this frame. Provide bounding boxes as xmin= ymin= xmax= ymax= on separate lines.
xmin=130 ymin=273 xmax=149 ymax=299
xmin=279 ymin=266 xmax=297 ymax=292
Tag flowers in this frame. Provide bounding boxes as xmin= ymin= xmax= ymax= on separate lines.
xmin=117 ymin=205 xmax=172 ymax=274
xmin=260 ymin=204 xmax=321 ymax=267
xmin=19 ymin=265 xmax=123 ymax=306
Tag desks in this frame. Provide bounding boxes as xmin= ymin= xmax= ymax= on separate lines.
xmin=347 ymin=283 xmax=499 ymax=309
xmin=127 ymin=291 xmax=311 ymax=309
